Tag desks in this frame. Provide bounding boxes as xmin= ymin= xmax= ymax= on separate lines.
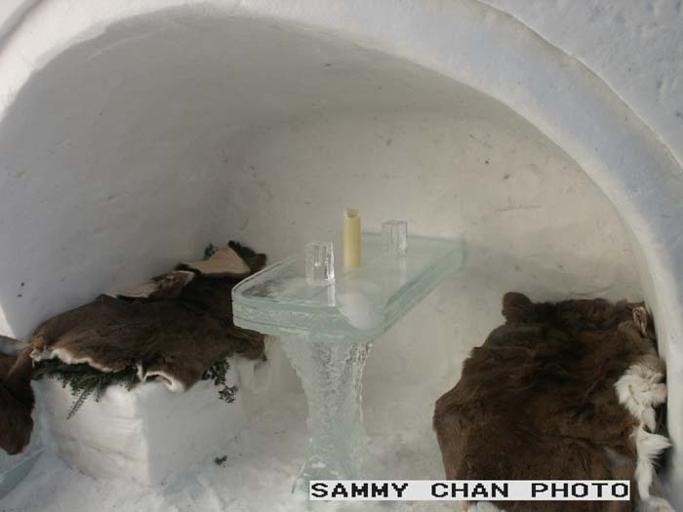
xmin=228 ymin=230 xmax=464 ymax=500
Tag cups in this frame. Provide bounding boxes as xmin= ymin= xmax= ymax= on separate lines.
xmin=379 ymin=219 xmax=408 ymax=257
xmin=303 ymin=239 xmax=334 ymax=283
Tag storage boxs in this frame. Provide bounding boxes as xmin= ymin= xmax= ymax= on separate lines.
xmin=34 ymin=336 xmax=301 ymax=488
